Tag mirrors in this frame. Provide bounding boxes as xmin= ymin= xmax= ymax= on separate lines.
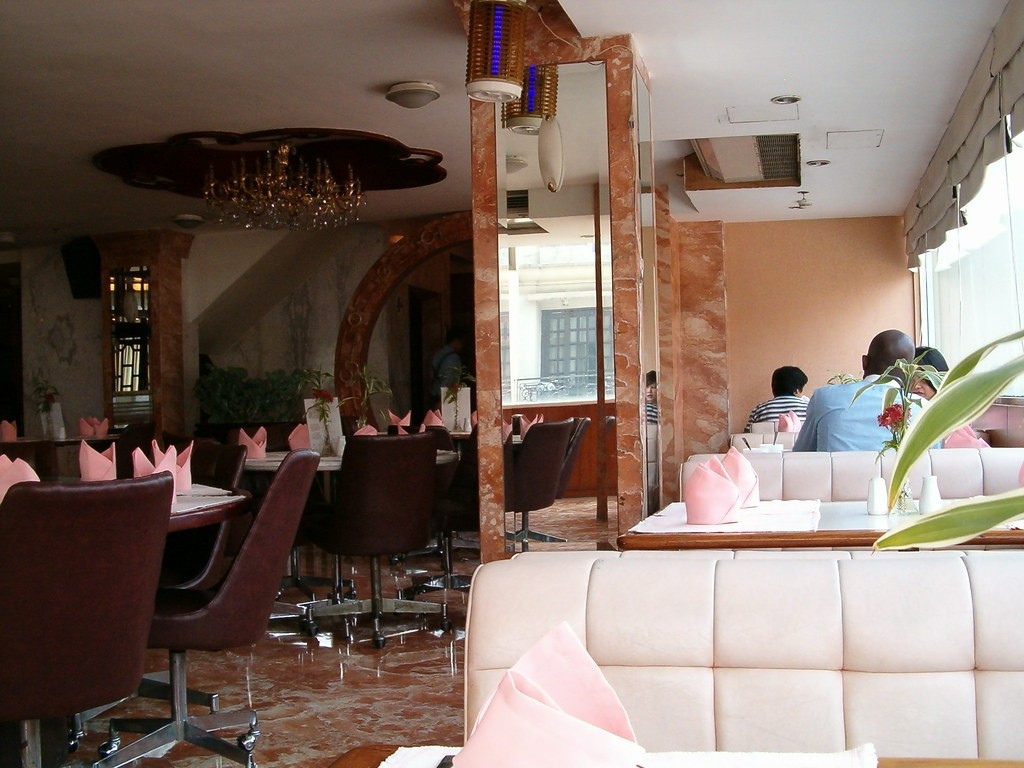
xmin=634 ymin=65 xmax=660 ymax=516
xmin=495 ymin=60 xmax=617 ymax=554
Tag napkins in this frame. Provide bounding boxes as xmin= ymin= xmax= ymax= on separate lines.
xmin=778 ymin=410 xmax=801 ymax=432
xmin=720 ymin=446 xmax=760 ymax=509
xmin=239 ymin=426 xmax=267 ymax=459
xmin=79 ymin=416 xmax=109 ymax=438
xmin=388 ymin=410 xmax=411 ymax=426
xmin=354 ymin=425 xmax=377 ymax=436
xmin=151 ymin=438 xmax=194 ymax=491
xmin=423 ymin=409 xmax=445 ymax=426
xmin=685 ymin=453 xmax=741 ymax=525
xmin=79 ymin=439 xmax=117 ymax=481
xmin=0 ymin=420 xmax=17 ymax=441
xmin=132 ymin=445 xmax=178 ymax=504
xmin=502 ymin=419 xmax=513 ymax=443
xmin=287 ymin=424 xmax=311 ymax=451
xmin=453 ymin=622 xmax=645 ymax=766
xmin=397 ymin=424 xmax=425 ymax=434
xmin=520 ymin=413 xmax=543 ymax=441
xmin=0 ymin=454 xmax=40 ymax=504
xmin=941 ymin=424 xmax=991 ymax=450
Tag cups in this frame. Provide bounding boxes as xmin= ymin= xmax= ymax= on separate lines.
xmin=760 ymin=444 xmax=784 ymax=453
xmin=742 ymin=448 xmax=760 ymax=454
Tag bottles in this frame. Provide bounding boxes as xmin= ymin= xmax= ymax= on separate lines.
xmin=866 ymin=477 xmax=889 ymax=516
xmin=919 ymin=475 xmax=943 ymax=516
xmin=463 ymin=418 xmax=471 ymax=432
xmin=59 ymin=427 xmax=66 ymax=439
xmin=335 ymin=435 xmax=346 ymax=456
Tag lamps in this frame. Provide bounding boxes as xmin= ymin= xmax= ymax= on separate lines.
xmin=506 ymin=156 xmax=528 ymax=175
xmin=538 ymin=114 xmax=563 ymax=191
xmin=465 ymin=0 xmax=530 ymax=103
xmin=501 ymin=62 xmax=557 ymax=133
xmin=173 ymin=214 xmax=207 ymax=230
xmin=387 ymin=82 xmax=440 ymax=110
xmin=92 ymin=128 xmax=448 ymax=231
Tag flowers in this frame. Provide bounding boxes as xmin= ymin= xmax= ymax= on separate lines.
xmin=337 ymin=363 xmax=393 ymax=426
xmin=438 ymin=361 xmax=478 ymax=430
xmin=296 ymin=364 xmax=334 ymax=447
xmin=25 ymin=378 xmax=59 ymax=427
xmin=851 ymin=345 xmax=947 ymax=464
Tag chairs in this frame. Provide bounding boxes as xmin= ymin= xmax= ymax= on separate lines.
xmin=503 ymin=414 xmax=592 ymax=553
xmin=0 ymin=419 xmax=479 ymax=768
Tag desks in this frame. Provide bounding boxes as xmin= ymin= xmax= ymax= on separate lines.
xmin=244 ymin=451 xmax=353 ymax=587
xmin=0 ymin=434 xmax=121 ymax=482
xmin=162 ymin=482 xmax=251 ymax=534
xmin=613 ymin=502 xmax=1023 ymax=548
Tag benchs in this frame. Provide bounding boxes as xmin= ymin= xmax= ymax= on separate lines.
xmin=460 ymin=414 xmax=1024 ymax=758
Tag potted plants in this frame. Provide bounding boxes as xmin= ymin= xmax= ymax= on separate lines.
xmin=191 ymin=363 xmax=309 ymax=444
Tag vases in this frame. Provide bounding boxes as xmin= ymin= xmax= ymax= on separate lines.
xmin=44 ymin=415 xmax=60 ymax=441
xmin=895 ymin=476 xmax=920 ymax=515
xmin=318 ymin=420 xmax=338 ymax=458
xmin=356 ymin=416 xmax=367 ymax=429
xmin=453 ymin=403 xmax=462 ymax=432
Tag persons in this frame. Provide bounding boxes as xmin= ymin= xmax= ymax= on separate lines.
xmin=646 ymin=371 xmax=658 ymax=424
xmin=430 ymin=325 xmax=467 ymax=413
xmin=793 ymin=331 xmax=940 ymax=452
xmin=908 ymin=347 xmax=984 ymax=448
xmin=744 ymin=366 xmax=811 ymax=433
